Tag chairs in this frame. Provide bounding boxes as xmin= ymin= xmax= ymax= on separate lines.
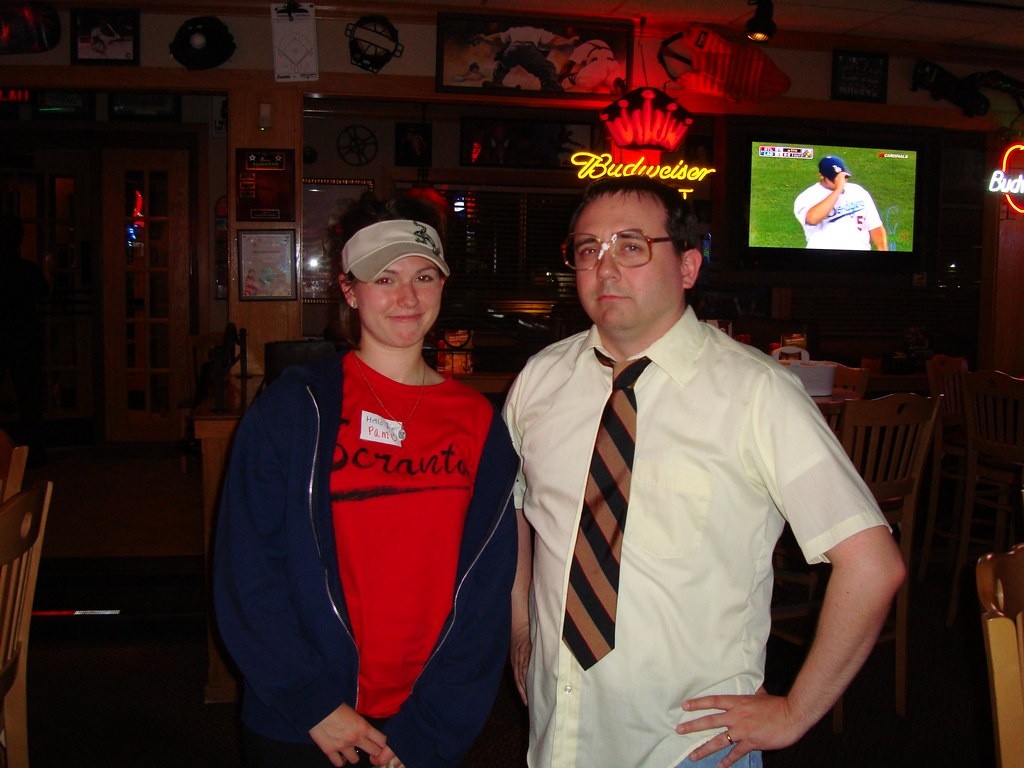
xmin=0 ymin=430 xmax=29 ymax=504
xmin=0 ymin=479 xmax=54 ymax=768
xmin=774 ymin=360 xmax=870 ymax=399
xmin=974 ymin=542 xmax=1024 ymax=768
xmin=766 ymin=391 xmax=942 ymax=731
xmin=947 ymin=365 xmax=1024 ymax=630
xmin=918 ymin=353 xmax=1014 ymax=579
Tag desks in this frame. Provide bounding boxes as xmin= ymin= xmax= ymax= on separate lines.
xmin=191 ymin=371 xmax=522 ymax=703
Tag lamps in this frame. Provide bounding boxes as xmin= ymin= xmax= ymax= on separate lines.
xmin=744 ymin=0 xmax=776 ymax=44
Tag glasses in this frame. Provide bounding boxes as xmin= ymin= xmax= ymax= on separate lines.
xmin=561 ymin=228 xmax=689 ymax=271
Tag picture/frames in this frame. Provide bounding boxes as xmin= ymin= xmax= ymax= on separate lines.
xmin=233 ymin=146 xmax=298 ymax=222
xmin=394 ymin=121 xmax=434 ymax=169
xmin=302 ymin=174 xmax=375 ymax=304
xmin=434 ymin=10 xmax=634 ymax=97
xmin=829 ymin=47 xmax=890 ymax=101
xmin=458 ymin=117 xmax=593 ymax=167
xmin=237 ymin=227 xmax=298 ymax=304
xmin=69 ymin=7 xmax=141 ymax=65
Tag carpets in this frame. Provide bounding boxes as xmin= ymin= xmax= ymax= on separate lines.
xmin=29 ymin=553 xmax=206 ymax=642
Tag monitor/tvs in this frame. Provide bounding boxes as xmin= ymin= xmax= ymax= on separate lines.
xmin=737 ymin=133 xmax=925 ymax=272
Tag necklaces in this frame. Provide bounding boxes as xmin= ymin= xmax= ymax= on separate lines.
xmin=353 ymin=345 xmax=428 ymax=440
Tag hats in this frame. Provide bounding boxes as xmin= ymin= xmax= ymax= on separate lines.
xmin=341 ymin=219 xmax=450 ymax=283
xmin=818 ymin=154 xmax=852 ymax=182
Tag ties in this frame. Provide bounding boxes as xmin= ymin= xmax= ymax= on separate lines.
xmin=561 ymin=346 xmax=652 ymax=670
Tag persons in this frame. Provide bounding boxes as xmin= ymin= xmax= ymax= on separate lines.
xmin=793 ymin=155 xmax=890 ymax=252
xmin=454 ymin=24 xmax=620 ymax=95
xmin=500 ymin=179 xmax=908 ymax=768
xmin=212 ymin=193 xmax=522 ymax=768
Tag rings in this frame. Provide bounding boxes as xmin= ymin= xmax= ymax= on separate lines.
xmin=725 ymin=731 xmax=734 ymax=746
